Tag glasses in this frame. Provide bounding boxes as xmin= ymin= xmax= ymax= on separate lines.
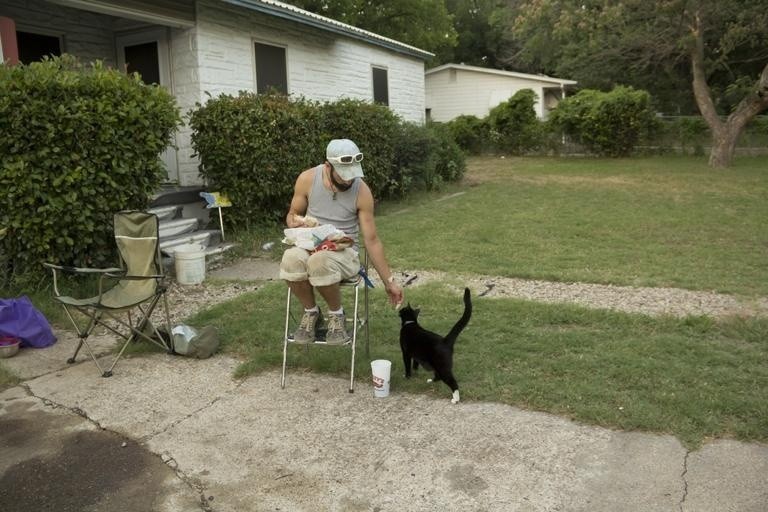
xmin=327 ymin=153 xmax=364 ymax=165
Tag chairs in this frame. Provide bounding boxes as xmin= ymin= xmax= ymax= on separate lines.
xmin=44 ymin=209 xmax=176 ymax=375
xmin=273 ymin=244 xmax=371 ymax=395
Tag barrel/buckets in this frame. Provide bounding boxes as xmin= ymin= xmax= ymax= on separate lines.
xmin=175 ymin=244 xmax=207 ymax=286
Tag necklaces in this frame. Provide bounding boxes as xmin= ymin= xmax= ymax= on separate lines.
xmin=325 ymin=165 xmax=340 ymax=201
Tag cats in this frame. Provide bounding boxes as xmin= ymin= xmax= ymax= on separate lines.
xmin=397 ymin=286 xmax=472 ymax=405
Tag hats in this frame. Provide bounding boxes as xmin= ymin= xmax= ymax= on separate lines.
xmin=325 ymin=139 xmax=365 ymax=182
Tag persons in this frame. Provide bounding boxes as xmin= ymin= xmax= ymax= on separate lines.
xmin=278 ymin=136 xmax=405 ymax=347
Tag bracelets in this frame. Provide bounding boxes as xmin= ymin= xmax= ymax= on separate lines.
xmin=383 ymin=277 xmax=395 ymax=286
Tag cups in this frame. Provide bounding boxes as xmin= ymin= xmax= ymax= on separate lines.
xmin=371 ymin=358 xmax=393 ymax=398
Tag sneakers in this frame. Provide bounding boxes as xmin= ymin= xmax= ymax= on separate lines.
xmin=326 ymin=308 xmax=350 ymax=346
xmin=294 ymin=306 xmax=323 ymax=345
